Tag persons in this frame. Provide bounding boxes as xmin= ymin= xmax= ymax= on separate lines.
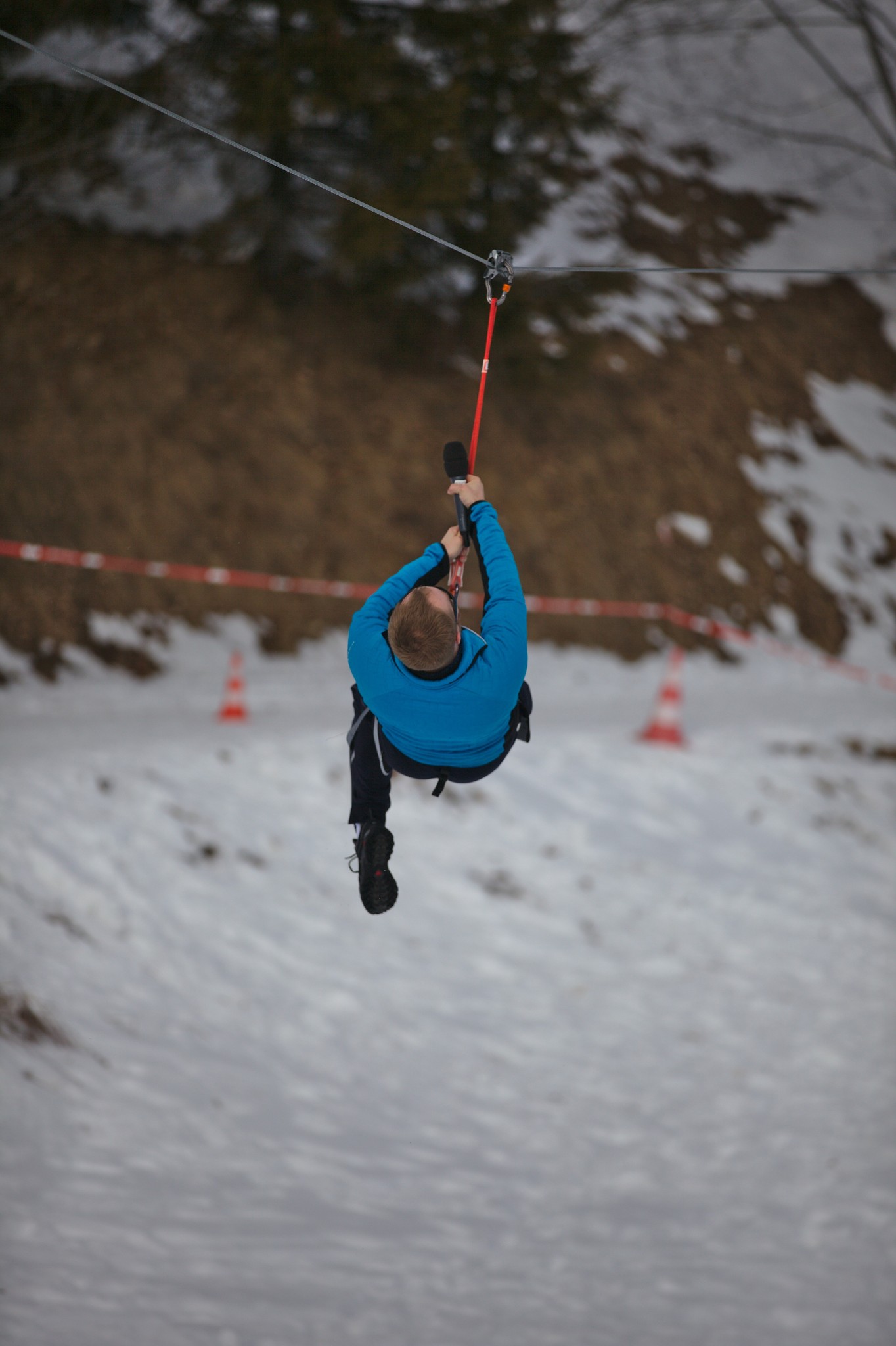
xmin=344 ymin=475 xmax=533 ymax=914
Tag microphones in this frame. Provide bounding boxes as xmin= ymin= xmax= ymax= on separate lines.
xmin=444 ymin=441 xmax=470 ymax=546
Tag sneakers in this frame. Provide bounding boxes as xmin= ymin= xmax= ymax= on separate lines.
xmin=346 ymin=819 xmax=398 ymax=914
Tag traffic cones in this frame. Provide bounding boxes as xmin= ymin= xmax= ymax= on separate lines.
xmin=636 ymin=647 xmax=691 ymax=747
xmin=217 ymin=651 xmax=251 ymax=724
